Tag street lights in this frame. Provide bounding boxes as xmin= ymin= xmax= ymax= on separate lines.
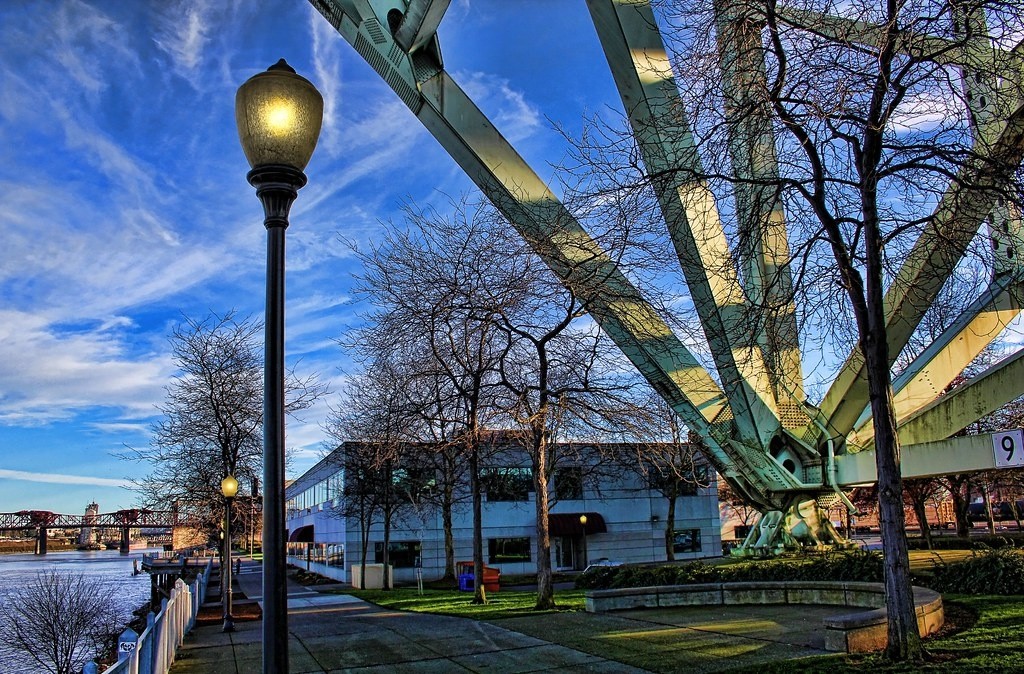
xmin=233 ymin=56 xmax=326 ymax=674
xmin=220 ymin=473 xmax=239 ymax=630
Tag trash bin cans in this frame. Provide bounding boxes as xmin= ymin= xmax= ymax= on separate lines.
xmin=163 ymin=544 xmax=173 ymax=551
